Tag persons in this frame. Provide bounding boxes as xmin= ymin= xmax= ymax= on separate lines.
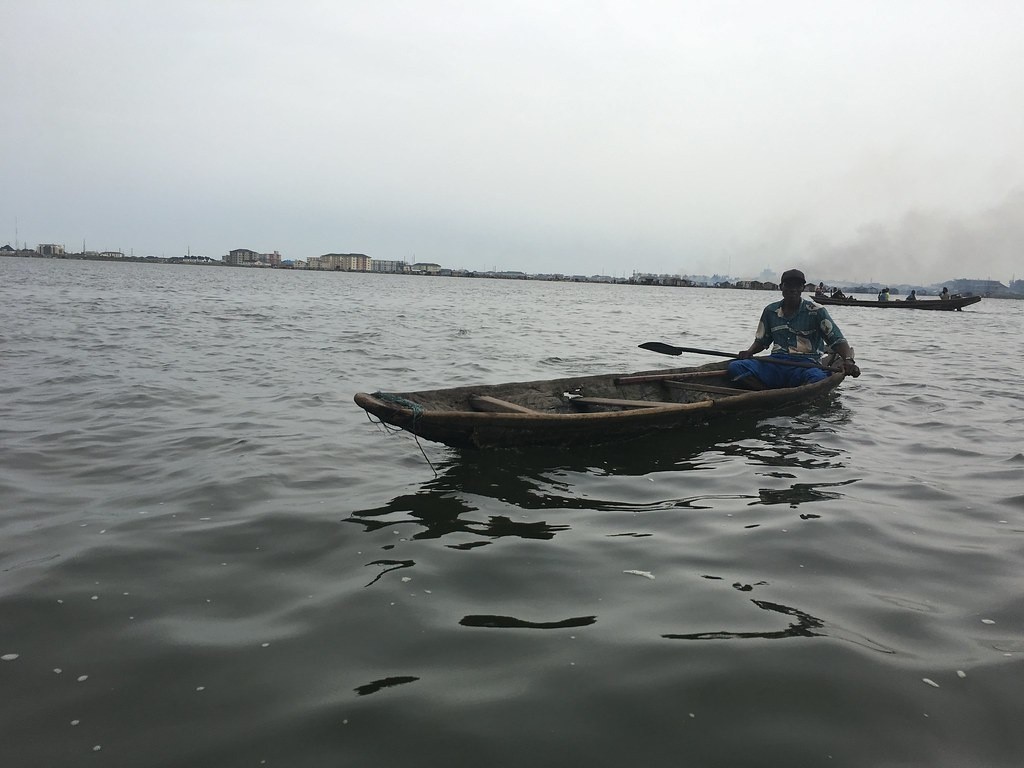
xmin=906 ymin=290 xmax=916 ymax=301
xmin=939 ymin=287 xmax=950 ymax=300
xmin=727 ymin=269 xmax=860 ymax=391
xmin=831 ymin=286 xmax=840 ymax=298
xmin=878 ymin=288 xmax=889 ymax=301
xmin=814 ymin=282 xmax=829 ymax=298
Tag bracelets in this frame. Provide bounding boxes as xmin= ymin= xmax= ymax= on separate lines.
xmin=844 ymin=357 xmax=855 ymax=364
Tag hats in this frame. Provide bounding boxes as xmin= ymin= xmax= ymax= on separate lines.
xmin=780 ymin=269 xmax=806 ymax=285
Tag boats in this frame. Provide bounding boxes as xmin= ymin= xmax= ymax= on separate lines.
xmin=353 ymin=346 xmax=847 ymax=451
xmin=809 ymin=295 xmax=982 ymax=311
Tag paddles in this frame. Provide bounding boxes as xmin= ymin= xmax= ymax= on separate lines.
xmin=637 ymin=341 xmax=845 ymax=371
